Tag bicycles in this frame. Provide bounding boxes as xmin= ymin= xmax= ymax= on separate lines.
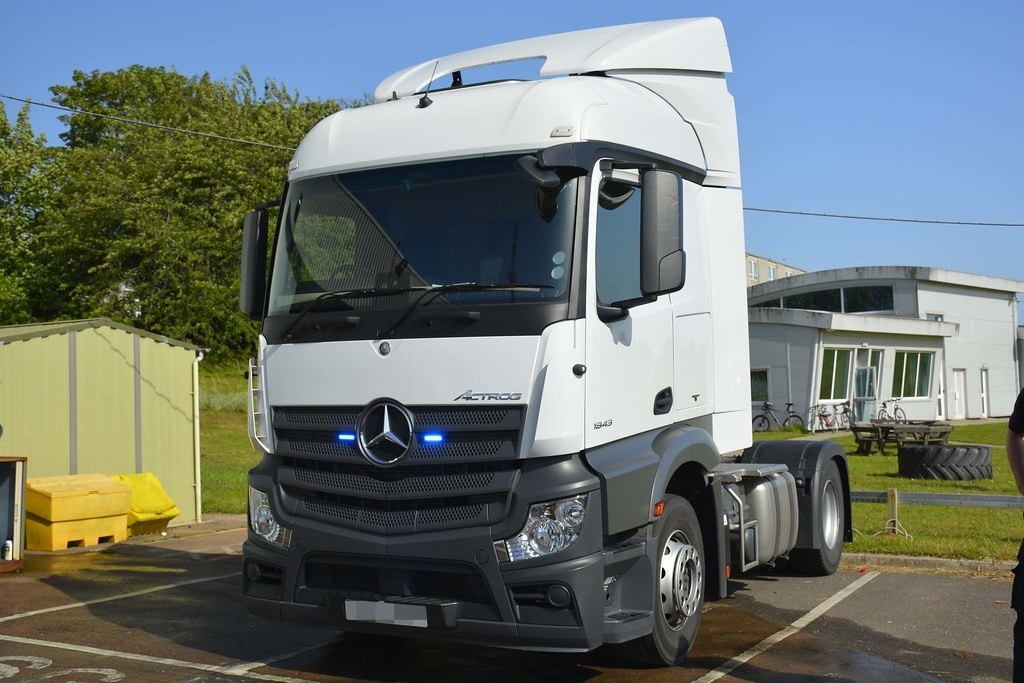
xmin=878 ymin=399 xmax=906 ymax=423
xmin=833 ymin=400 xmax=857 ymax=432
xmin=752 ymin=402 xmax=803 ymax=431
xmin=806 ymin=404 xmax=839 ymax=434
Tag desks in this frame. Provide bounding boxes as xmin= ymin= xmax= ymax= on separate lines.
xmin=874 ymin=424 xmax=922 ymax=442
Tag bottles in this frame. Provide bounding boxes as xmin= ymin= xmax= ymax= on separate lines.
xmin=4 ymin=536 xmax=12 ymax=561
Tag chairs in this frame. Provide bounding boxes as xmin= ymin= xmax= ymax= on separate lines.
xmin=485 ymin=182 xmax=565 ymax=287
xmin=371 ymin=174 xmax=465 ymax=286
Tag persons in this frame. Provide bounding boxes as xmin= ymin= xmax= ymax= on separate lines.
xmin=1006 ymin=388 xmax=1024 ymax=683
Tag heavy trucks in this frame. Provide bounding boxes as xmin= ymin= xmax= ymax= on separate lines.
xmin=237 ymin=15 xmax=852 ymax=667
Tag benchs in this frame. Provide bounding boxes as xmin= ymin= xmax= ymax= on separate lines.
xmin=849 ymin=419 xmax=954 ymax=456
